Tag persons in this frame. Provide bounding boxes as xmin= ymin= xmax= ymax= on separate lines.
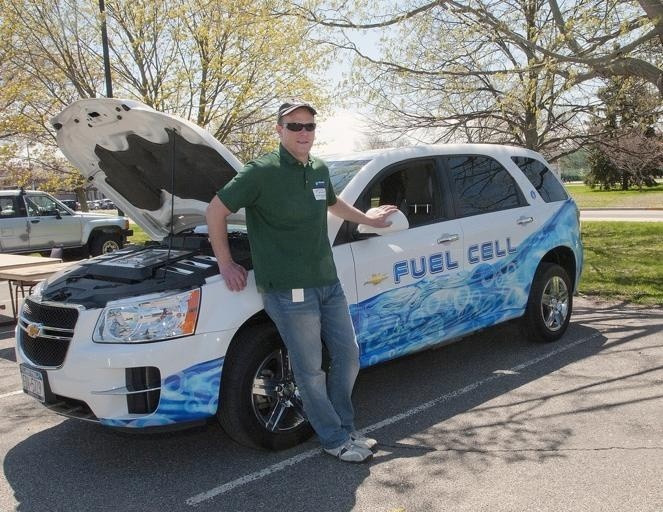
xmin=205 ymin=102 xmax=399 ymax=462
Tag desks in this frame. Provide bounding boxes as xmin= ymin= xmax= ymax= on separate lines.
xmin=0 ymin=254 xmax=87 ymax=327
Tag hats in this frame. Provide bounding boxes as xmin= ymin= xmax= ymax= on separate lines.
xmin=278 ymin=102 xmax=317 ymax=120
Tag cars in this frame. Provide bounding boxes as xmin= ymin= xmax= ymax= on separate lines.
xmin=0 ymin=190 xmax=133 ymax=262
xmin=15 ymin=98 xmax=583 ymax=450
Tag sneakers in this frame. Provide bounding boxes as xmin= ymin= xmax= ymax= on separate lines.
xmin=324 ymin=431 xmax=377 ymax=463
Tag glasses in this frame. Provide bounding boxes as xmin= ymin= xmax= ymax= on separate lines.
xmin=279 ymin=123 xmax=316 ymax=130
